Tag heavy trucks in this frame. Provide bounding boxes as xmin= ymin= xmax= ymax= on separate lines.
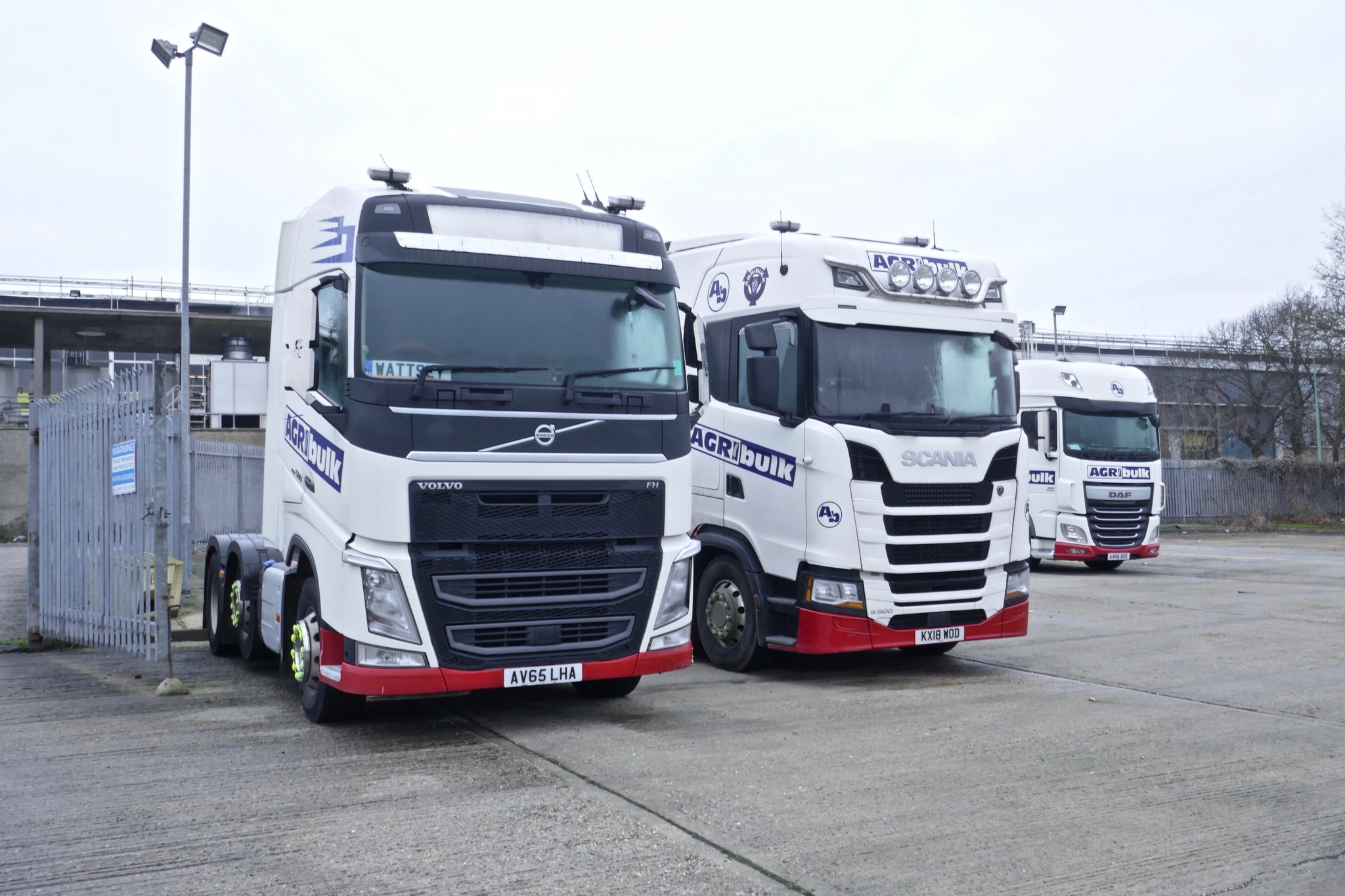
xmin=1013 ymin=358 xmax=1166 ymax=572
xmin=661 ymin=218 xmax=1031 ymax=674
xmin=202 ymin=162 xmax=701 ymax=725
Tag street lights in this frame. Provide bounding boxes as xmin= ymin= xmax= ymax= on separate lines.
xmin=1049 ymin=306 xmax=1066 ymax=355
xmin=1265 ymin=338 xmax=1325 ymax=521
xmin=150 ymin=23 xmax=230 ymax=594
